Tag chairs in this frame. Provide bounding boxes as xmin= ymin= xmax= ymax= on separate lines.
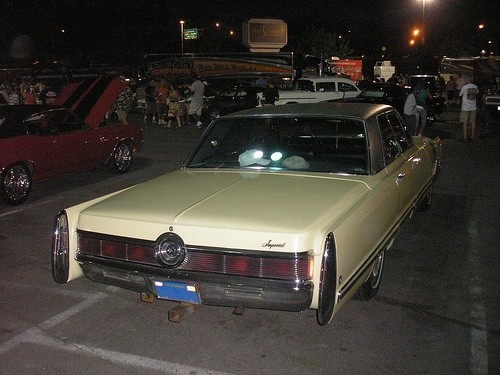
xmin=247 ymin=128 xmax=283 ymax=166
xmin=337 ymin=145 xmax=367 ymax=172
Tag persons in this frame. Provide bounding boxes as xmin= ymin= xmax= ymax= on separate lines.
xmin=112 ymin=74 xmax=204 ymax=132
xmin=0 ymin=77 xmax=60 ymax=106
xmin=254 ymin=73 xmax=280 ymax=107
xmin=371 ymin=72 xmax=479 ymax=143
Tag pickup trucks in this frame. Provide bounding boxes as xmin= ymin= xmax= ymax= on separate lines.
xmin=481 ymin=92 xmax=500 ymax=118
xmin=272 ymin=75 xmax=386 ymax=105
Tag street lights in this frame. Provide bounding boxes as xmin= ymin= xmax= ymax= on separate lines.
xmin=408 ymin=0 xmax=431 ymax=56
xmin=179 ymin=18 xmax=187 ymax=52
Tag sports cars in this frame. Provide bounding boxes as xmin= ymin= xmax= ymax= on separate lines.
xmin=49 ymin=97 xmax=444 ymax=328
xmin=0 ymin=71 xmax=145 ymax=208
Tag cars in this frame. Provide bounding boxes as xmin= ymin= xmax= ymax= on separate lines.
xmin=193 ymin=83 xmax=276 ymax=121
xmin=324 ymin=80 xmax=445 ymax=128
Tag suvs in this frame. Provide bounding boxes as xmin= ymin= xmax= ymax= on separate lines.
xmin=403 ymin=74 xmax=446 ymax=114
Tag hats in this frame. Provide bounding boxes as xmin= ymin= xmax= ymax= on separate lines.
xmin=413 ymin=86 xmax=421 ymax=92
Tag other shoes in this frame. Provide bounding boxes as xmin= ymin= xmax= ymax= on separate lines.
xmin=163 ymin=124 xmax=172 ymax=129
xmin=151 ymin=121 xmax=157 ymax=124
xmin=458 ymin=138 xmax=469 ymax=143
xmin=158 ymin=120 xmax=162 ymax=125
xmin=467 ymin=136 xmax=475 ymax=142
xmin=174 ymin=125 xmax=181 ymax=131
xmin=162 ymin=119 xmax=167 ymax=124
xmin=194 ymin=121 xmax=202 ymax=129
xmin=143 ymin=117 xmax=147 ymax=124
